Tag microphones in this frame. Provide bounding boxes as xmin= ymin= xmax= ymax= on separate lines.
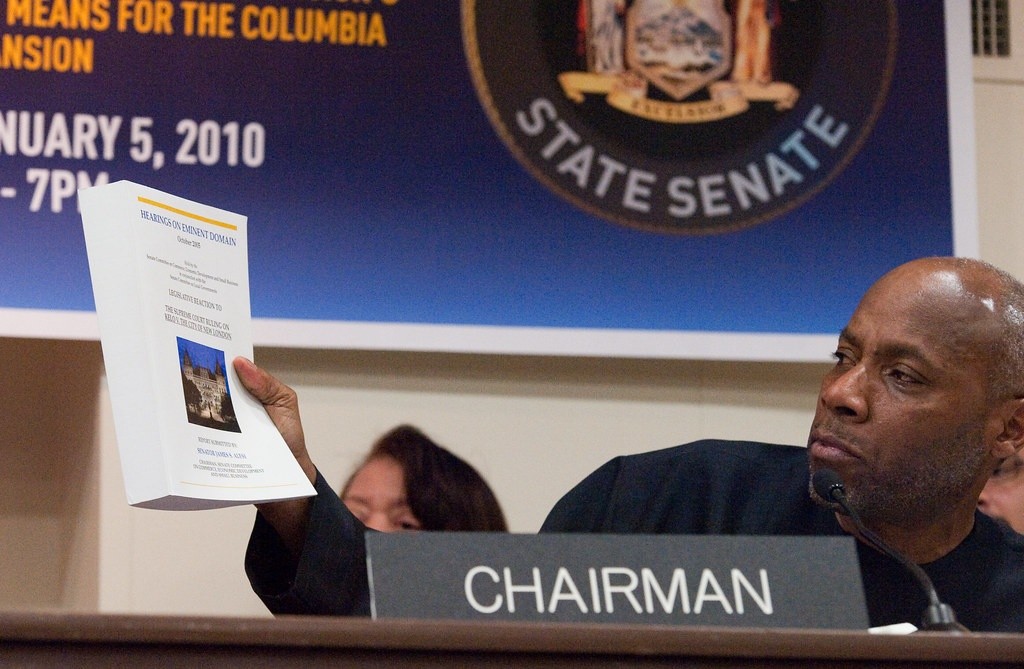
xmin=812 ymin=468 xmax=971 ymax=635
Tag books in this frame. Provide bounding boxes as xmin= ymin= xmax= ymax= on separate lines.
xmin=75 ymin=178 xmax=319 ymax=513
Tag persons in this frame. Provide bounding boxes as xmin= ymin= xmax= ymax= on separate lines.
xmin=232 ymin=255 xmax=1022 ymax=633
